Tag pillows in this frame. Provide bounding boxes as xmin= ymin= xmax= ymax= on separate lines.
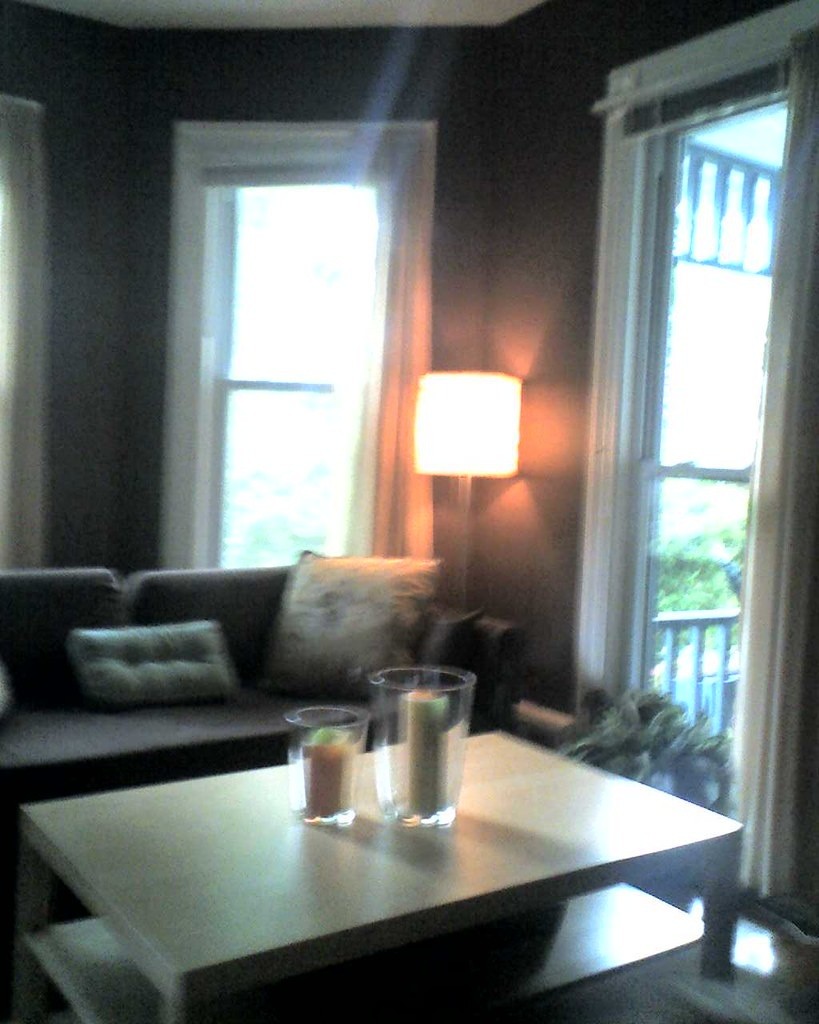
xmin=261 ymin=550 xmax=446 ymax=699
xmin=65 ymin=618 xmax=247 ymax=709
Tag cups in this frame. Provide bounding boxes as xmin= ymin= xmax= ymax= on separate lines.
xmin=282 ymin=704 xmax=369 ymax=824
xmin=367 ymin=666 xmax=477 ymax=832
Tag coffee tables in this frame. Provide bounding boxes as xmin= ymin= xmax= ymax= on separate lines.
xmin=11 ymin=730 xmax=744 ymax=1023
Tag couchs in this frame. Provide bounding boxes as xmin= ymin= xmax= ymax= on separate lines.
xmin=0 ymin=559 xmax=529 ymax=877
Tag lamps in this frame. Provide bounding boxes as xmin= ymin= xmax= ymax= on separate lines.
xmin=413 ymin=368 xmax=522 ymax=616
